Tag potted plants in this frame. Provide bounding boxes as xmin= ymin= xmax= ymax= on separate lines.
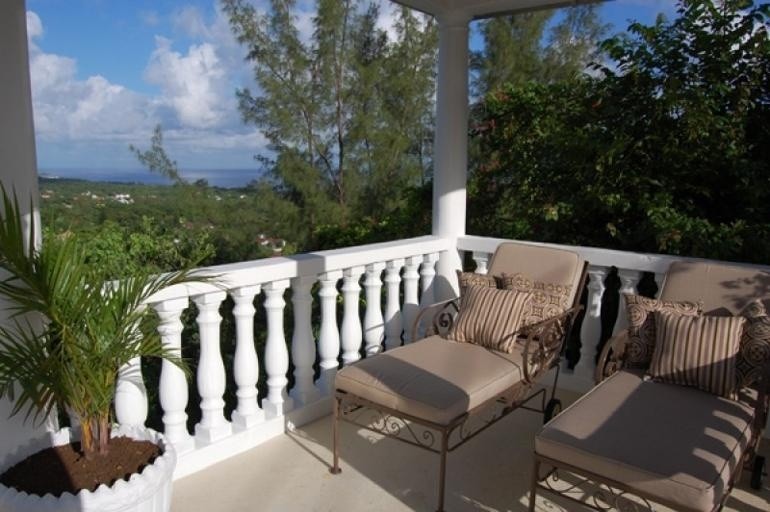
xmin=0 ymin=187 xmax=228 ymax=512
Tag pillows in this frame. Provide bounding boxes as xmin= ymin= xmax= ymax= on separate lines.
xmin=455 ymin=269 xmax=502 ymax=310
xmin=642 ymin=308 xmax=747 ymax=402
xmin=622 ymin=291 xmax=704 ymax=371
xmin=502 ymin=271 xmax=574 ymax=339
xmin=447 ymin=284 xmax=534 ymax=355
xmin=730 ymin=297 xmax=770 ymax=388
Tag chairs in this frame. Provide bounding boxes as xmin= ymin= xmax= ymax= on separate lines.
xmin=332 ymin=240 xmax=588 ymax=512
xmin=529 ymin=261 xmax=770 ymax=511
